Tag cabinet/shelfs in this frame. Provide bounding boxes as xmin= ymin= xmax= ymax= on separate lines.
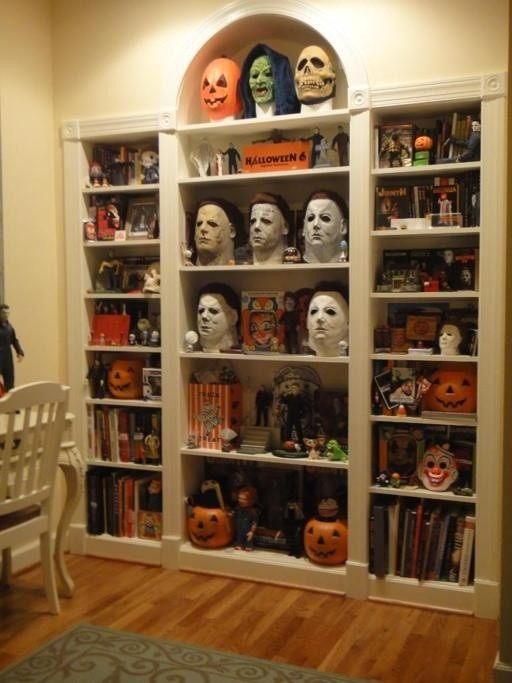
xmin=61 ymin=69 xmax=512 ymax=621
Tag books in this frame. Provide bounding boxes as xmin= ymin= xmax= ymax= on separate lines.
xmin=244 ymin=428 xmax=272 ymax=436
xmin=240 ymin=443 xmax=267 ymax=453
xmin=374 ymin=178 xmax=480 ymax=229
xmin=242 ymin=435 xmax=271 ymax=440
xmin=369 ymin=496 xmax=476 ymax=588
xmin=242 ymin=439 xmax=269 ymax=445
xmin=85 ymin=402 xmax=162 ymax=541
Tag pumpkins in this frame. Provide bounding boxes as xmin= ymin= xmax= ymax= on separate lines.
xmin=199 ymin=58 xmax=242 ymax=120
xmin=415 ymin=135 xmax=433 ymax=151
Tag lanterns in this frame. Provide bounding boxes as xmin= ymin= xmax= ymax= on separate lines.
xmin=198 ymin=52 xmax=244 ymax=121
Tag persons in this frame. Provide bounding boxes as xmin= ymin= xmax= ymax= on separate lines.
xmin=83 ymin=356 xmax=106 ymax=399
xmin=188 ymin=115 xmax=482 ymax=178
xmin=196 ymin=282 xmax=240 ymax=352
xmin=253 ymin=383 xmax=273 ymax=427
xmin=280 ymin=290 xmax=300 ymax=354
xmin=247 ymin=189 xmax=293 ymax=263
xmin=296 ymin=279 xmax=349 ymax=356
xmin=436 ymin=319 xmax=469 ymax=354
xmin=193 ymin=196 xmax=238 ymax=265
xmin=0 ymin=304 xmax=25 ymax=413
xmin=81 ymin=148 xmax=162 ymax=348
xmin=223 ymin=484 xmax=265 ymax=551
xmin=241 ymin=42 xmax=290 ymax=116
xmin=280 ymin=493 xmax=307 ymax=559
xmin=302 ymin=188 xmax=348 ymax=262
xmin=279 ymin=381 xmax=306 ymax=452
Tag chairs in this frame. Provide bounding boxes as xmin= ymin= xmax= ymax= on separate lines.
xmin=0 ymin=382 xmax=71 ymax=615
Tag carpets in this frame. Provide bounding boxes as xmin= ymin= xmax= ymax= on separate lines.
xmin=0 ymin=623 xmax=385 ymax=683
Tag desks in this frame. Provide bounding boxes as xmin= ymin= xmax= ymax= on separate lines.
xmin=0 ymin=412 xmax=87 ymax=599
